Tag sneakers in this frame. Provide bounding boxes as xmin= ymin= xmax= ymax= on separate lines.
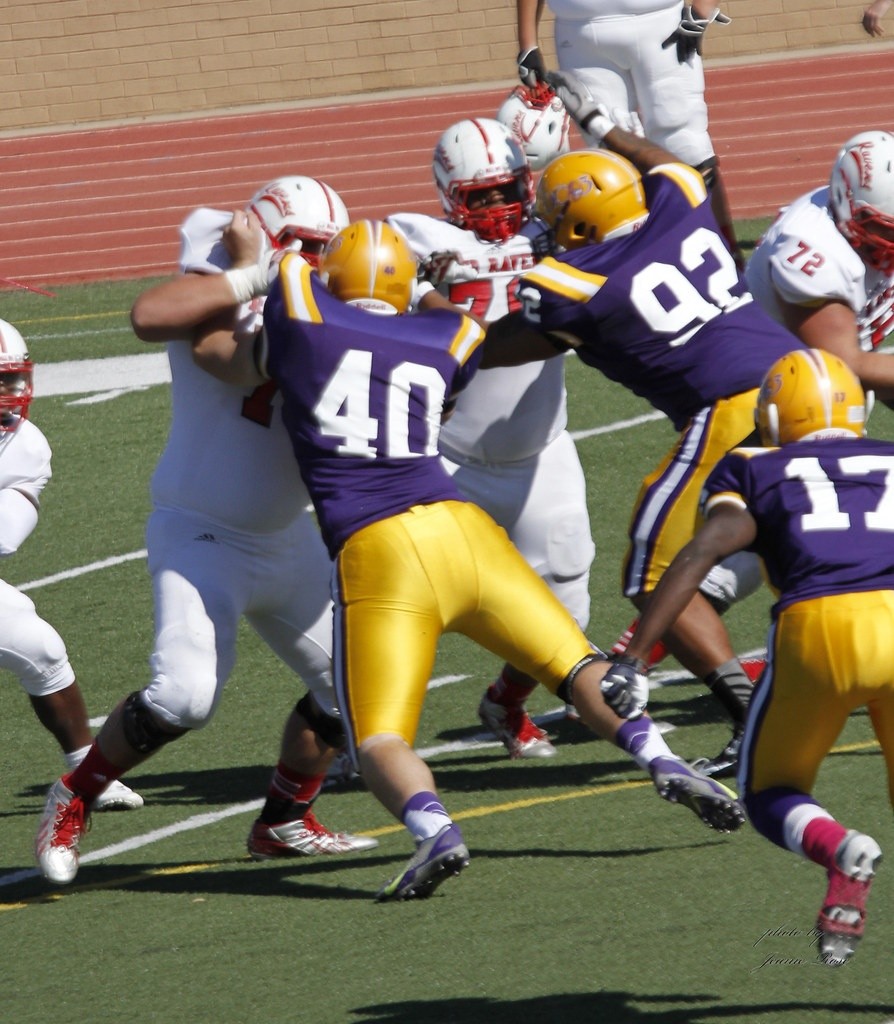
xmin=697 ymin=723 xmax=747 ymax=780
xmin=35 ymin=776 xmax=95 ymax=884
xmin=92 ymin=779 xmax=144 ymax=810
xmin=374 ymin=822 xmax=471 ymax=902
xmin=479 ymin=686 xmax=557 ymax=759
xmin=817 ymin=828 xmax=883 ymax=967
xmin=247 ymin=811 xmax=378 ymax=861
xmin=564 ymin=703 xmax=589 ymax=728
xmin=647 ymin=755 xmax=747 ymax=834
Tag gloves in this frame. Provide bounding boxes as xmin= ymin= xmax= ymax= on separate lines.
xmin=661 ymin=6 xmax=731 ymax=63
xmin=226 ymin=237 xmax=303 ymax=306
xmin=516 ymin=46 xmax=558 ymax=93
xmin=545 ymin=70 xmax=602 ymax=138
xmin=600 ymin=657 xmax=649 ymax=722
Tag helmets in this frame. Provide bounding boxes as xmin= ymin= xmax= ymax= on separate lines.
xmin=497 ymin=80 xmax=571 ymax=171
xmin=432 ymin=117 xmax=537 ymax=241
xmin=830 ymin=130 xmax=894 ymax=278
xmin=245 ymin=174 xmax=349 ymax=269
xmin=0 ymin=316 xmax=33 ymax=431
xmin=528 ymin=147 xmax=650 ymax=262
xmin=755 ymin=347 xmax=865 ymax=448
xmin=320 ymin=219 xmax=420 ymax=317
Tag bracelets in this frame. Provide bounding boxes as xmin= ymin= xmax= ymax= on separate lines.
xmin=411 ymin=281 xmax=435 ymax=308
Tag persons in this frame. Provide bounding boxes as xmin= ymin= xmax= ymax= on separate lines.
xmin=612 ymin=129 xmax=894 ymax=680
xmin=323 ymin=117 xmax=596 ymax=787
xmin=191 ymin=208 xmax=746 ymax=905
xmin=862 ymin=0 xmax=894 ymax=37
xmin=0 ymin=318 xmax=143 ymax=810
xmin=517 ymin=0 xmax=747 ymax=272
xmin=601 ymin=348 xmax=894 ymax=965
xmin=34 ymin=173 xmax=378 ymax=884
xmin=472 ymin=69 xmax=808 ymax=779
